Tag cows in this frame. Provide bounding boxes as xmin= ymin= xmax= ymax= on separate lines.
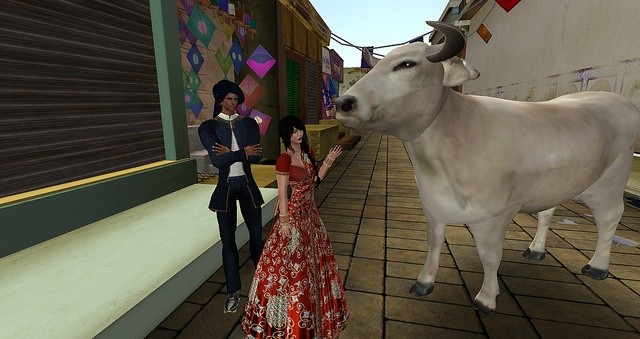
xmin=334 ymin=20 xmax=640 ymax=314
